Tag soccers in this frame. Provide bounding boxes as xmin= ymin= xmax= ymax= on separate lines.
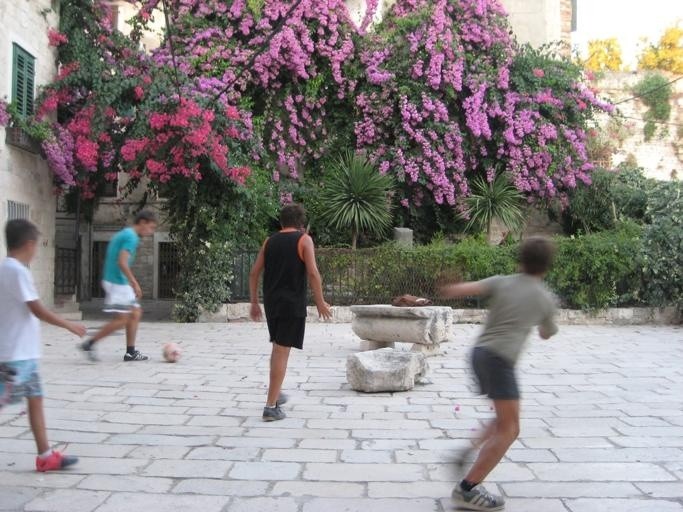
xmin=164 ymin=343 xmax=180 ymax=362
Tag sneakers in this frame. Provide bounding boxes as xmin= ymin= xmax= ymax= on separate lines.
xmin=124 ymin=351 xmax=148 ymax=361
xmin=263 ymin=394 xmax=288 ymax=420
xmin=80 ymin=343 xmax=100 ymax=362
xmin=36 ymin=450 xmax=79 ymax=472
xmin=448 ymin=482 xmax=507 ymax=512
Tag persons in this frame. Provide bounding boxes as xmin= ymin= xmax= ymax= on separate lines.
xmin=435 ymin=235 xmax=559 ymax=512
xmin=249 ymin=204 xmax=333 ymax=422
xmin=0 ymin=218 xmax=87 ymax=472
xmin=77 ymin=210 xmax=157 ymax=365
